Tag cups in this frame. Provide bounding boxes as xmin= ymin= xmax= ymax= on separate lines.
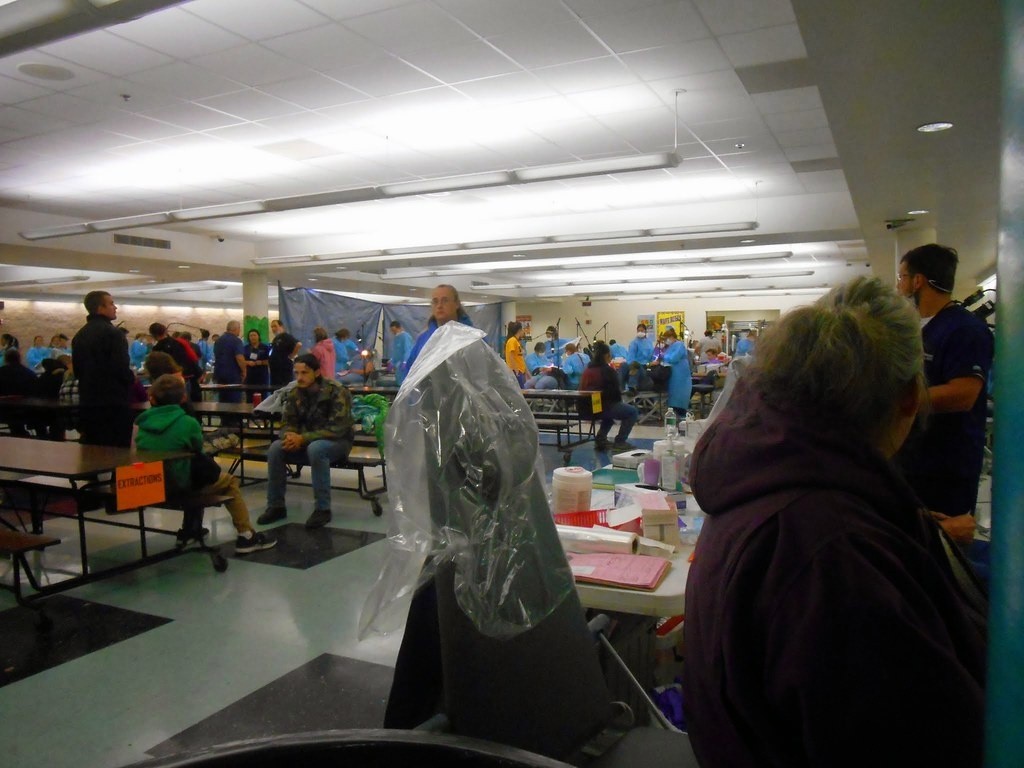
xmin=253 ymin=394 xmax=262 ymax=407
xmin=644 ymin=459 xmax=661 ymax=486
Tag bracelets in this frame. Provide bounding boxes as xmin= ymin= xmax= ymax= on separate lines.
xmin=261 ymin=359 xmax=263 ymax=365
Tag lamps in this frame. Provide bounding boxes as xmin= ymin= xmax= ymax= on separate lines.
xmin=631 ymin=255 xmax=709 ymax=270
xmin=547 ymin=228 xmax=647 ymax=242
xmin=18 ymin=220 xmax=91 ymax=243
xmin=624 ymin=276 xmax=682 ymax=283
xmin=469 ymin=284 xmax=517 ymax=289
xmin=170 ymin=197 xmax=269 ymax=225
xmin=570 ymin=280 xmax=625 ymax=285
xmin=378 ymin=269 xmax=434 ymax=280
xmin=381 ymin=242 xmax=463 ymax=254
xmin=515 ymin=149 xmax=685 ymax=189
xmin=708 ymin=249 xmax=793 ymax=264
xmin=561 ymin=259 xmax=632 ymax=271
xmin=518 ymin=281 xmax=570 ymax=287
xmin=491 ymin=262 xmax=561 ymax=276
xmin=748 ymin=270 xmax=815 ymax=280
xmin=370 ymin=167 xmax=513 ymax=196
xmin=464 ymin=235 xmax=551 ymax=250
xmin=312 ymin=248 xmax=384 ymax=259
xmin=247 ymin=253 xmax=315 ymax=266
xmin=436 ymin=267 xmax=492 ymax=277
xmin=88 ymin=211 xmax=172 ymax=232
xmin=648 ymin=217 xmax=763 ymax=241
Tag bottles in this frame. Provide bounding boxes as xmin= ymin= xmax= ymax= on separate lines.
xmin=664 ymin=408 xmax=677 ymax=438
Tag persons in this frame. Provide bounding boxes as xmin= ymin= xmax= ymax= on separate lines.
xmin=0 ymin=321 xmax=374 ymax=441
xmin=685 ymin=242 xmax=996 ymax=768
xmin=387 ymin=321 xmax=414 ymax=387
xmin=401 ymin=284 xmax=489 ymax=382
xmin=134 ymin=374 xmax=278 ymax=552
xmin=257 ymin=353 xmax=354 ymax=528
xmin=71 ymin=291 xmax=151 ymax=447
xmin=505 ymin=321 xmax=758 ymax=427
xmin=575 ymin=344 xmax=638 ymax=450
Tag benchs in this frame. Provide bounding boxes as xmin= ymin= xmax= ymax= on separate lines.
xmin=532 ymin=409 xmax=613 ymax=440
xmin=0 ymin=526 xmax=64 ymax=607
xmin=535 ymin=418 xmax=578 ymax=450
xmin=214 ymin=444 xmax=392 ymax=500
xmin=0 ymin=471 xmax=235 ymax=550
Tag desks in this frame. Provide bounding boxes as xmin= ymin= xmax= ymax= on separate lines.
xmin=0 ymin=434 xmax=198 ymax=579
xmin=546 ymin=463 xmax=705 ymax=731
xmin=519 ymin=388 xmax=605 ymax=448
xmin=350 ymin=385 xmax=400 ymax=398
xmin=620 ymin=391 xmax=668 ymax=424
xmin=129 ymin=383 xmax=285 ymax=487
xmin=199 ymin=377 xmax=289 ymax=395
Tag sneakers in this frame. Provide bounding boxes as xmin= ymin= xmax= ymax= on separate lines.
xmin=232 ymin=528 xmax=279 ymax=555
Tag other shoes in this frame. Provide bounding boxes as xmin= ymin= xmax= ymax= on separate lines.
xmin=305 ymin=505 xmax=334 ymax=530
xmin=610 ymin=441 xmax=639 ymax=452
xmin=593 ymin=436 xmax=614 ymax=447
xmin=255 ymin=504 xmax=289 ymax=527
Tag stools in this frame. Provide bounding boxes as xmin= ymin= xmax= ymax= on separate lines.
xmin=691 ymin=382 xmax=716 ymax=418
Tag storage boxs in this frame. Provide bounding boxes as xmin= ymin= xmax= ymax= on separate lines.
xmin=669 ymin=494 xmax=688 ymax=515
xmin=552 ymin=507 xmax=643 ymax=532
